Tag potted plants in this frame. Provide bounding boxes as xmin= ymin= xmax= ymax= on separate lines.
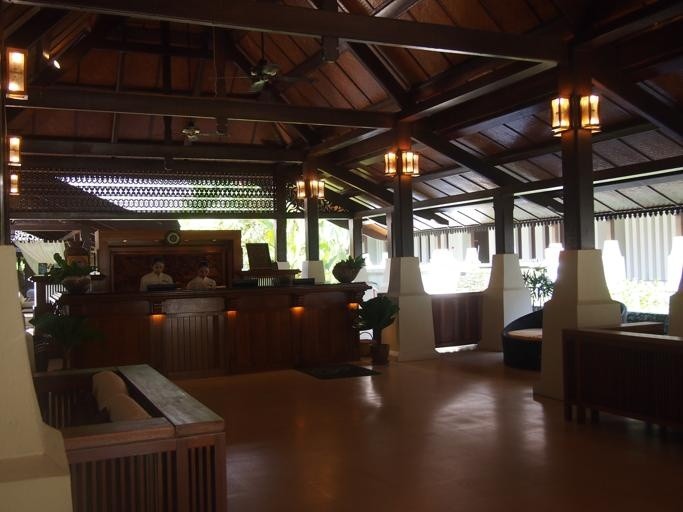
xmin=347 ymin=295 xmax=400 ymax=364
xmin=332 ymin=252 xmax=366 ymax=283
xmin=48 ymin=252 xmax=97 ymax=294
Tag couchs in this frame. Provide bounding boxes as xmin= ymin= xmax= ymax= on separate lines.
xmin=500 ymin=300 xmax=626 ymax=372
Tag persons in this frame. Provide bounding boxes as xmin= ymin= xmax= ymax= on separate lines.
xmin=186 ymin=260 xmax=216 ymax=290
xmin=139 ymin=258 xmax=174 ymax=292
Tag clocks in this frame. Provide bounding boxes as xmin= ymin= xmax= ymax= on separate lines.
xmin=164 ymin=230 xmax=180 ymax=247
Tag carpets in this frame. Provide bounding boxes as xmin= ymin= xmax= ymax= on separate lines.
xmin=292 ymin=364 xmax=381 ymax=380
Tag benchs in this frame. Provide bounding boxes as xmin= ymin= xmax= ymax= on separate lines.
xmin=31 ymin=361 xmax=229 ymax=510
xmin=559 ymin=321 xmax=682 ymax=433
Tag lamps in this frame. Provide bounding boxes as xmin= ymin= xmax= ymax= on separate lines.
xmin=4 ymin=46 xmax=29 ymax=100
xmin=295 ymin=178 xmax=325 ymax=200
xmin=150 ymin=116 xmax=230 ymax=146
xmin=208 ymin=32 xmax=319 ymax=93
xmin=550 ymin=93 xmax=602 ymax=138
xmin=5 ymin=134 xmax=23 ymax=167
xmin=383 ymin=149 xmax=420 ymax=178
xmin=7 ymin=173 xmax=20 ymax=196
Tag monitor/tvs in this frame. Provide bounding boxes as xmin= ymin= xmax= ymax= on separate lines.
xmin=232 ymin=279 xmax=258 ymax=289
xmin=147 ymin=284 xmax=176 ymax=291
